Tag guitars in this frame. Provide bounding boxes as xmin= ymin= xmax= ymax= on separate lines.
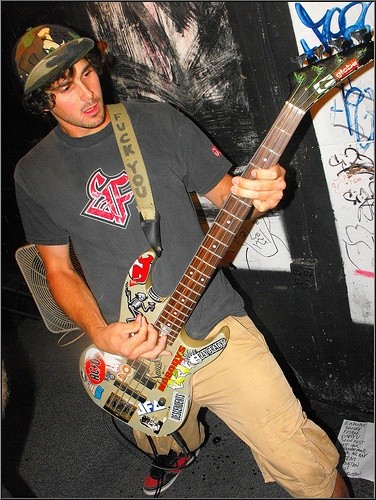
xmin=79 ymin=28 xmax=375 ymax=437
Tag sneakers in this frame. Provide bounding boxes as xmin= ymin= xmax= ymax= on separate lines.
xmin=143 ymin=448 xmax=201 ymax=498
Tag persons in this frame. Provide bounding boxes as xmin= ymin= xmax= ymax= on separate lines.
xmin=15 ymin=24 xmax=353 ymax=498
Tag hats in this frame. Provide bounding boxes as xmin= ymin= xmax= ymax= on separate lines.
xmin=12 ymin=24 xmax=95 ymax=96
